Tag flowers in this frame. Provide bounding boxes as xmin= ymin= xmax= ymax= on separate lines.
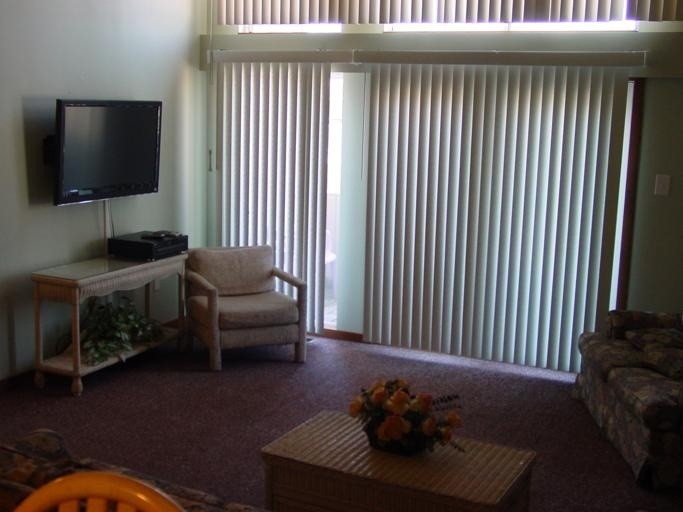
xmin=346 ymin=377 xmax=468 ymax=454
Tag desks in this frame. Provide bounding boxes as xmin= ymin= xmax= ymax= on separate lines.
xmin=29 ymin=252 xmax=188 ymax=397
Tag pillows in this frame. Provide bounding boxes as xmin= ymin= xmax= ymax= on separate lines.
xmin=625 ymin=327 xmax=682 ymax=379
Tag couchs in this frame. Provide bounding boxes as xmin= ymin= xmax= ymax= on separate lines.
xmin=0 ymin=427 xmax=267 ymax=511
xmin=570 ymin=308 xmax=682 ymax=490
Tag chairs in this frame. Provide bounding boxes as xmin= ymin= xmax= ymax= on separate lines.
xmin=184 ymin=244 xmax=307 ymax=371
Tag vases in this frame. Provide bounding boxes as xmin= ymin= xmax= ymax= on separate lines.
xmin=367 ymin=427 xmax=425 ymax=457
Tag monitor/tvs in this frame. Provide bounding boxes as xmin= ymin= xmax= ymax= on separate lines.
xmin=54 ymin=99 xmax=163 ymax=206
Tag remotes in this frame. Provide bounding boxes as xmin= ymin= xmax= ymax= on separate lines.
xmin=141 ymin=232 xmax=164 ymax=238
xmin=156 ymin=230 xmax=182 ymax=237
